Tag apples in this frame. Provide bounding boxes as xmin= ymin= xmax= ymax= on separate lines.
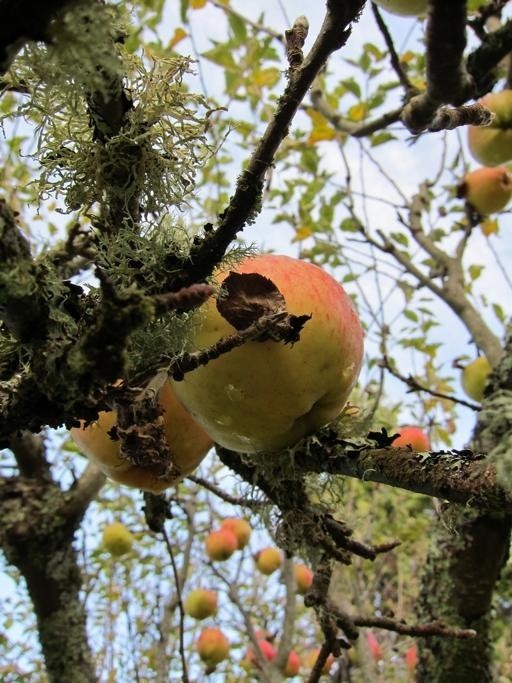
xmin=248 ymin=638 xmax=274 ymax=667
xmin=406 ymin=644 xmax=419 ymax=670
xmin=370 ymin=0 xmax=433 ymax=18
xmin=104 ymin=522 xmax=134 ymax=557
xmin=465 ymin=167 xmax=512 ymax=215
xmin=467 ymin=88 xmax=512 ymax=166
xmin=183 ymin=590 xmax=218 ymax=619
xmin=169 ymin=254 xmax=363 ymax=455
xmin=366 ymin=631 xmax=384 ymax=662
xmin=253 ymin=548 xmax=284 ymax=574
xmin=284 ymin=650 xmax=301 ymax=678
xmin=308 ymin=649 xmax=334 ymax=673
xmin=70 ymin=373 xmax=216 ymax=495
xmin=389 ymin=427 xmax=431 ymax=451
xmin=294 ymin=565 xmax=314 ymax=593
xmin=219 ymin=517 xmax=252 ymax=551
xmin=196 ymin=629 xmax=230 ymax=664
xmin=206 ymin=530 xmax=238 ymax=561
xmin=460 ymin=357 xmax=490 ymax=402
